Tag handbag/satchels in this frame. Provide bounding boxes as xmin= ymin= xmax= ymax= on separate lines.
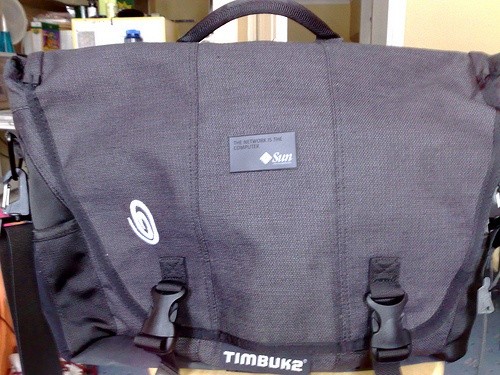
xmin=3 ymin=0 xmax=500 ymax=374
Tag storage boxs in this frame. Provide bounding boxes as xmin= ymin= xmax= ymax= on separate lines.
xmin=70 ymin=15 xmax=178 ymax=52
